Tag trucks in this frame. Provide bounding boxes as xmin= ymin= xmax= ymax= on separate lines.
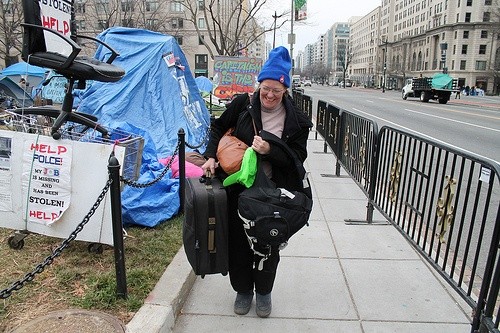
xmin=401 ymin=74 xmax=461 ymax=104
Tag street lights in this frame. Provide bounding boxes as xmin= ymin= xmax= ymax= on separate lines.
xmin=373 ymin=36 xmax=388 ymax=93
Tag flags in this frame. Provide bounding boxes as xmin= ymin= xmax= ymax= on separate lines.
xmin=295 ymin=0 xmax=308 ymax=21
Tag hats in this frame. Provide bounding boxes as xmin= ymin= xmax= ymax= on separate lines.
xmin=257 ymin=46 xmax=292 ymax=89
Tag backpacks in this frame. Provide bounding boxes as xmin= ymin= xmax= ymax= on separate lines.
xmin=236 ymin=131 xmax=314 ymax=271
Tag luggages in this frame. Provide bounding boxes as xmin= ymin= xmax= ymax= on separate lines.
xmin=182 ymin=172 xmax=228 ymax=278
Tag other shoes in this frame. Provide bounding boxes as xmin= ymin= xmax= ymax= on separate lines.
xmin=256 ymin=292 xmax=273 ymax=318
xmin=234 ymin=292 xmax=254 ymax=315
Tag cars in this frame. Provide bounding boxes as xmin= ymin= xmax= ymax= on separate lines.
xmin=337 ymin=78 xmax=352 ymax=87
xmin=291 ymin=75 xmax=324 ymax=87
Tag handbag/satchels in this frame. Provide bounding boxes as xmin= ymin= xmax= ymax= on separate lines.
xmin=217 ymin=127 xmax=248 ymax=176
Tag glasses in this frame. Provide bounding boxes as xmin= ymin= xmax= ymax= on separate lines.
xmin=261 ymin=86 xmax=285 ymax=95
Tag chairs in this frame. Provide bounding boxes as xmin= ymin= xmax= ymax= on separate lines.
xmin=20 ymin=0 xmax=125 ymax=142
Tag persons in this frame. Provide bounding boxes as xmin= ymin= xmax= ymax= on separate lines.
xmin=465 ymin=85 xmax=470 ymax=96
xmin=455 ymin=86 xmax=461 ymax=99
xmin=202 ymin=46 xmax=313 ymax=318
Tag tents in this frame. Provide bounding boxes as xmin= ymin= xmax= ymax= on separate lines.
xmin=0 ymin=62 xmax=51 ymax=90
